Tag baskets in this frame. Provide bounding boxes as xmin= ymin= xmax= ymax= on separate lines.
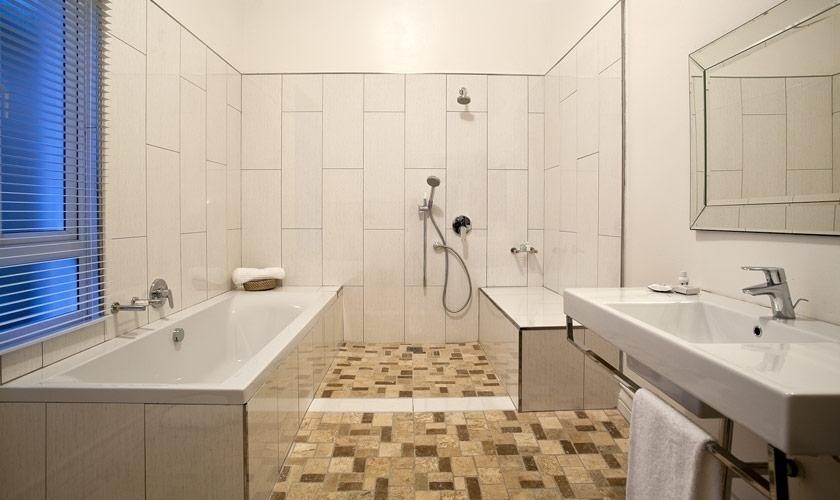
xmin=244 ymin=279 xmax=275 ymax=291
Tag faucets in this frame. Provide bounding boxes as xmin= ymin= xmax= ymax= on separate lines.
xmin=131 ymin=297 xmax=165 ymax=307
xmin=741 ymin=266 xmax=809 ymax=320
xmin=520 ymin=240 xmax=532 ymax=253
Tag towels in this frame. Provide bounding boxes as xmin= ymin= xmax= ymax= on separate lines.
xmin=626 ymin=387 xmax=727 ymax=498
xmin=232 ymin=267 xmax=287 ymax=286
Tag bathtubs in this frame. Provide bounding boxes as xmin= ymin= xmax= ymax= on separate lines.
xmin=0 ymin=291 xmax=338 ymax=403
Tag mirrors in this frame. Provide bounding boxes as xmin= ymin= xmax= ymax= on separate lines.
xmin=689 ymin=0 xmax=838 ymax=237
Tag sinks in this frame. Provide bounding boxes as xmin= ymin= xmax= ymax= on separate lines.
xmin=602 ymin=300 xmax=836 ymax=455
